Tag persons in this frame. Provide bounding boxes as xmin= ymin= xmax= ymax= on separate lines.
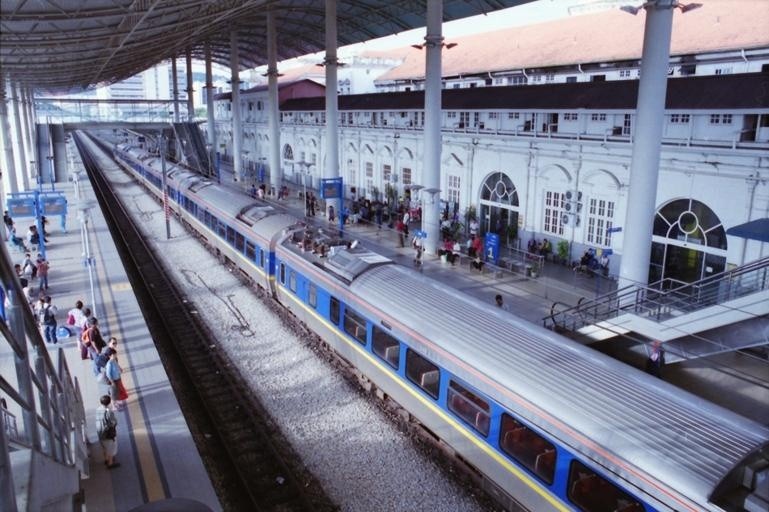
xmin=344 ymin=196 xmax=421 ymax=247
xmin=305 ymin=191 xmax=316 ymax=216
xmin=252 ymin=183 xmax=288 ymax=201
xmin=441 ymin=205 xmax=485 ymax=273
xmin=413 ymin=232 xmax=427 ymax=265
xmin=493 ymin=292 xmax=510 ymax=311
xmin=2 ymin=210 xmax=130 ymax=470
xmin=527 ymin=237 xmax=551 ymax=263
xmin=328 ymin=206 xmax=334 ymax=224
xmin=495 ymin=220 xmax=501 ymax=233
xmin=581 ymin=248 xmax=608 ymax=278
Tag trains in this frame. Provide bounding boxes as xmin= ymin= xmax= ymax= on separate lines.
xmin=86 ymin=128 xmax=769 ymax=511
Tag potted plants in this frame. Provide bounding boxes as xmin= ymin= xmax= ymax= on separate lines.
xmin=558 ymin=239 xmax=571 ymax=265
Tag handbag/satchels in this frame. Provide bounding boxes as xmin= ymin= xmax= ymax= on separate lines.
xmin=68 ymin=315 xmax=75 ymax=325
xmin=104 ymin=373 xmax=112 ymax=385
xmin=107 ymin=427 xmax=116 ymax=439
xmin=81 ymin=328 xmax=94 ymax=347
xmin=44 ymin=315 xmax=55 ymax=325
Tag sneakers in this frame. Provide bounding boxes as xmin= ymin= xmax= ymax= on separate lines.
xmin=104 ymin=458 xmax=120 ymax=469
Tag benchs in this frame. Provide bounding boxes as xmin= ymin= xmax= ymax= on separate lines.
xmin=575 ymin=258 xmax=606 ymax=276
xmin=355 ymin=325 xmax=617 ymax=511
xmin=369 ymin=201 xmax=556 ymax=280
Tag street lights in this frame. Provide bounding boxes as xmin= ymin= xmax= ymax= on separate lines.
xmin=294 ymin=159 xmax=316 ymax=218
xmin=407 ymin=184 xmax=444 ymax=277
xmin=63 ymin=132 xmax=102 ymax=327
xmin=155 ymin=133 xmax=267 ymax=200
